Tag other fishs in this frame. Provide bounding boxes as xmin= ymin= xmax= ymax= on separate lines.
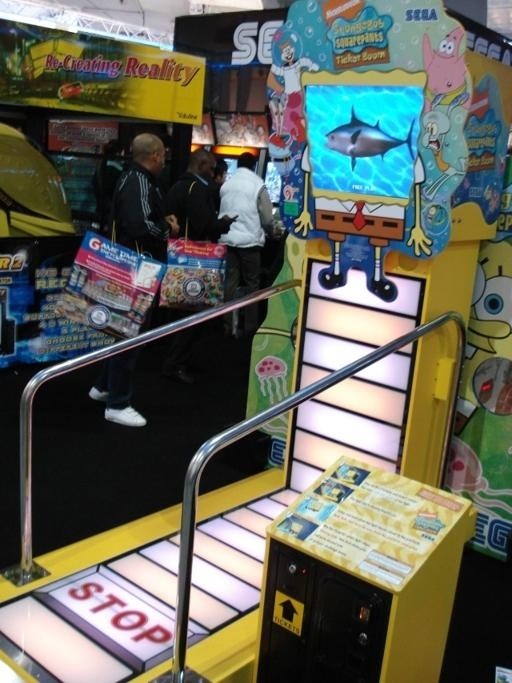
xmin=324 ymin=105 xmax=417 ymax=171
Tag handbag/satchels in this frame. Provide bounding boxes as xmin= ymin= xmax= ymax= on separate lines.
xmin=55 ymin=218 xmax=165 ymax=340
xmin=158 ymin=215 xmax=228 ymax=311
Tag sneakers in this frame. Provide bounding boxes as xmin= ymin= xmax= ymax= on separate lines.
xmin=104 ymin=406 xmax=146 ymax=426
xmin=88 ymin=386 xmax=109 ymax=401
